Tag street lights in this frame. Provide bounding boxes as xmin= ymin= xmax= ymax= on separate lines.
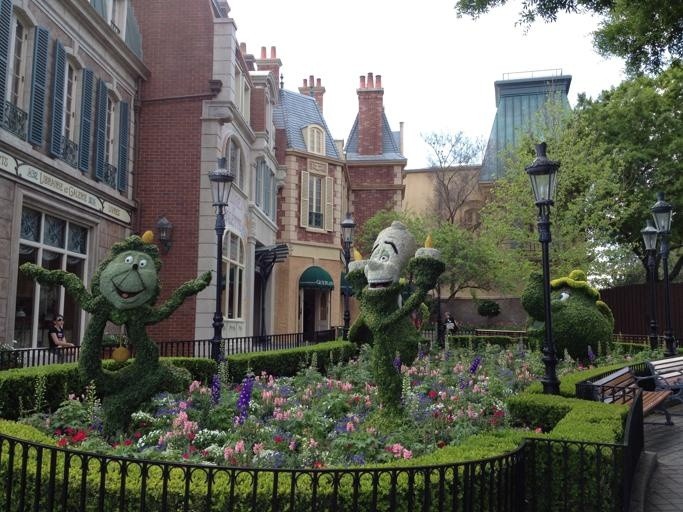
xmin=522 ymin=141 xmax=562 ymax=395
xmin=338 ymin=211 xmax=355 ymax=326
xmin=640 ymin=197 xmax=675 ymax=358
xmin=209 ymin=156 xmax=234 ymax=359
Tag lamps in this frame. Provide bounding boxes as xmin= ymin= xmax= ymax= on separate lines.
xmin=157 ymin=216 xmax=172 ymax=251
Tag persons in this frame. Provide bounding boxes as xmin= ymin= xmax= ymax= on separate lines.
xmin=442 ymin=312 xmax=458 ymax=336
xmin=47 ymin=313 xmax=76 ymax=359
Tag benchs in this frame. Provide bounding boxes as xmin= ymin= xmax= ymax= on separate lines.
xmin=588 ymin=367 xmax=673 ymax=429
xmin=650 ymin=356 xmax=683 ymax=403
